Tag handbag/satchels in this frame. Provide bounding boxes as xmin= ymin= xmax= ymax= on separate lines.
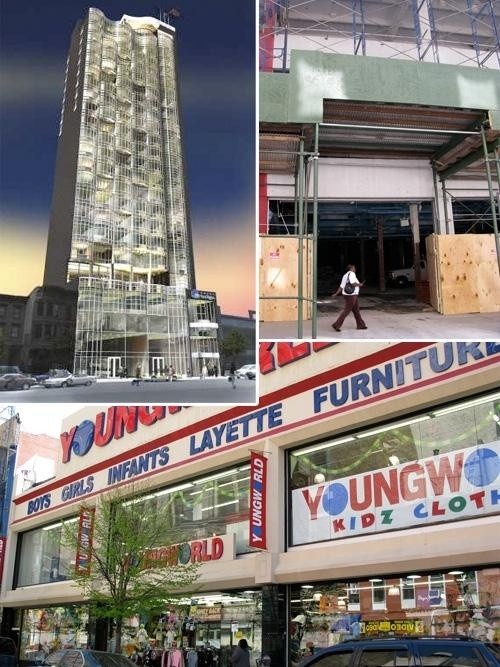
xmin=345 ymin=283 xmax=355 ymax=294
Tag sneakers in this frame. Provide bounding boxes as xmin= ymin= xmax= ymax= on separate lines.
xmin=332 ymin=324 xmax=341 ymax=332
xmin=360 ymin=326 xmax=367 ymax=329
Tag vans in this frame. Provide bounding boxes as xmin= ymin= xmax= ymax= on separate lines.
xmin=0 ymin=635 xmax=19 ymax=667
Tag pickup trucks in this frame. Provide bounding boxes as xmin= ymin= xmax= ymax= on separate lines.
xmin=389 ymin=259 xmax=429 ymax=285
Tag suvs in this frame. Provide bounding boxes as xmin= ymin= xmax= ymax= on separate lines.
xmin=293 ymin=631 xmax=500 ymax=667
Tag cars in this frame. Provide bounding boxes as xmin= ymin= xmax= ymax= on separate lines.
xmin=34 ymin=368 xmax=96 ymax=387
xmin=234 ymin=364 xmax=256 ymax=380
xmin=0 ymin=374 xmax=36 ymax=390
xmin=33 ymin=648 xmax=141 ymax=667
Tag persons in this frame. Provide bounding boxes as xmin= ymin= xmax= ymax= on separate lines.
xmin=228 ymin=639 xmax=252 ymax=667
xmin=463 ymin=584 xmax=477 ymax=608
xmin=136 ymin=624 xmax=148 ymax=642
xmin=330 ymin=264 xmax=368 ymax=332
xmin=116 ymin=363 xmax=239 ymax=388
xmin=468 ymin=613 xmax=492 ymax=641
